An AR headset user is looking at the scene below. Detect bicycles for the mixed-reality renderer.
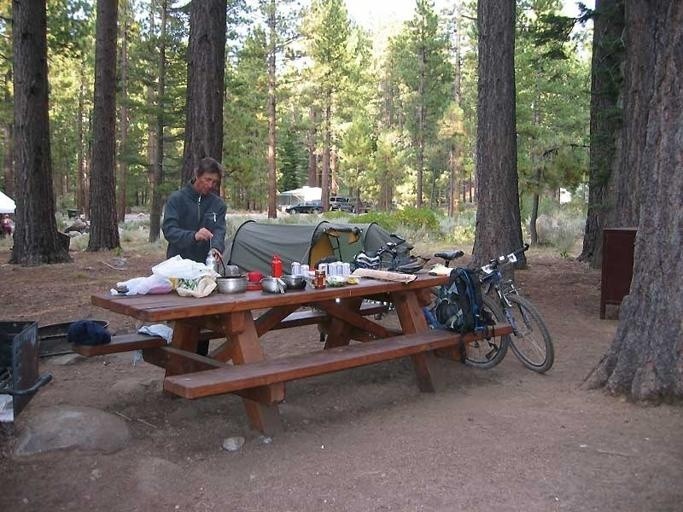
[317,233,555,374]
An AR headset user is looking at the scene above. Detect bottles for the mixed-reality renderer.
[271,256,282,277]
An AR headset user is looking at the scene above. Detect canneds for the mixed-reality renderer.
[313,270,326,290]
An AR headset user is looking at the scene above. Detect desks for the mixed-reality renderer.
[92,271,451,438]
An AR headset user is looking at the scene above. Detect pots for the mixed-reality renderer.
[216,276,247,294]
[260,276,287,294]
[281,274,307,290]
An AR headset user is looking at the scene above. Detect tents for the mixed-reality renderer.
[218,219,415,277]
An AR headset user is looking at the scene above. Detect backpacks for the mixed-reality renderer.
[435,267,484,330]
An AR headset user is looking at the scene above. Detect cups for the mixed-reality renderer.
[301,263,309,274]
[290,261,300,274]
[317,261,351,276]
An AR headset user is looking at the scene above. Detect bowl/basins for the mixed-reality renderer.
[344,274,360,284]
[325,277,347,287]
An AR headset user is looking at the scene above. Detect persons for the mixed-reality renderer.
[162,156,228,355]
[3,215,13,235]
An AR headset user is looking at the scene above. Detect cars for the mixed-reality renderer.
[286,196,374,215]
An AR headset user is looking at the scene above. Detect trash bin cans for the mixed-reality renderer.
[600,229,639,320]
[66,209,80,219]
